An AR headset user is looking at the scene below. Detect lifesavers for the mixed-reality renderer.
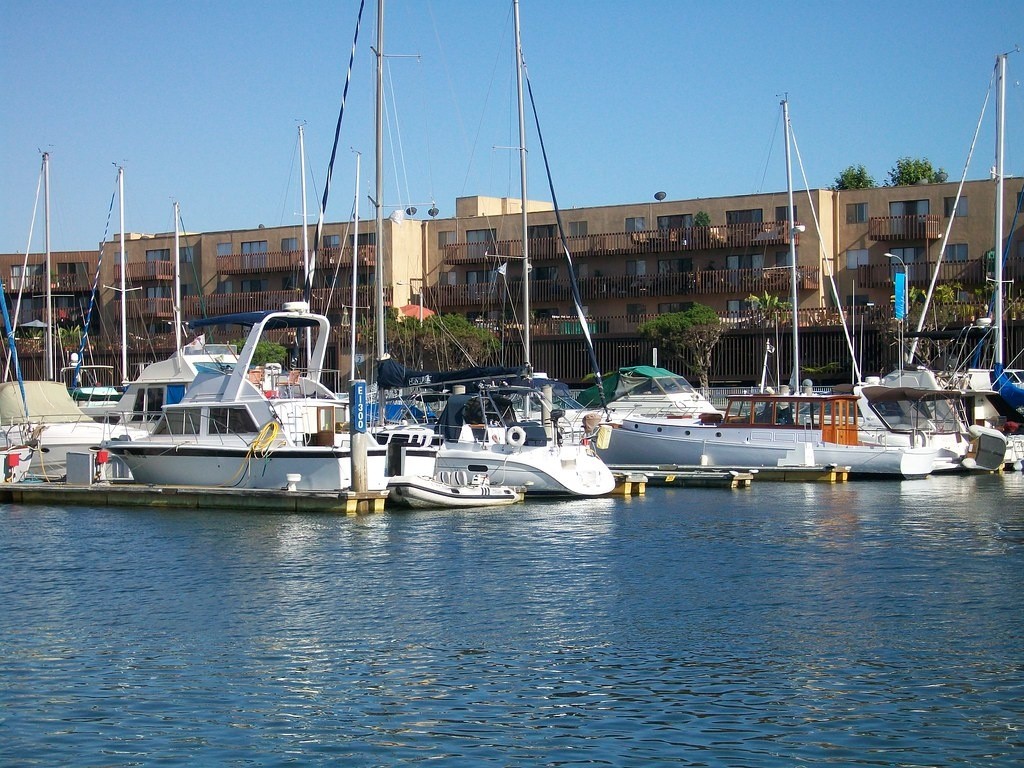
[507,426,526,446]
[910,430,928,447]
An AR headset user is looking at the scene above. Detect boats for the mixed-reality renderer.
[1,0,1024,510]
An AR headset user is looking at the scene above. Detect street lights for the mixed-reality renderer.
[884,252,909,335]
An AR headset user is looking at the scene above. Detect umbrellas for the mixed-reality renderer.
[20,319,53,331]
